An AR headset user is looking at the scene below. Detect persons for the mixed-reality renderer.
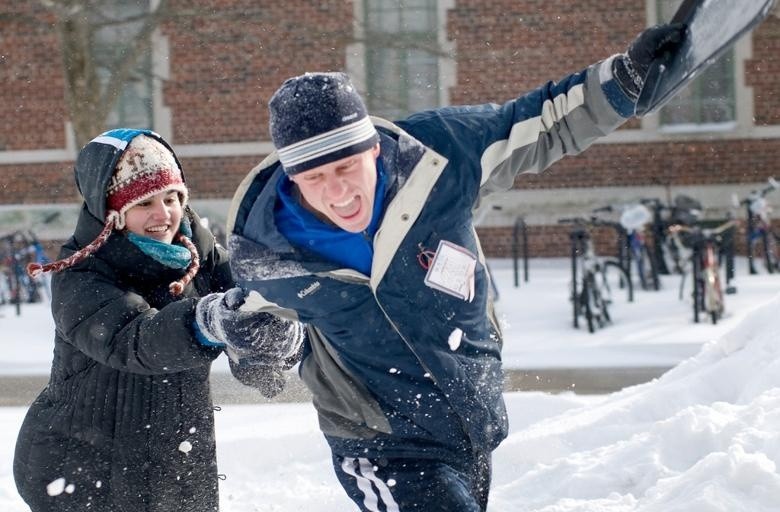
[224,0,767,511]
[620,198,693,276]
[1,229,54,304]
[11,125,305,511]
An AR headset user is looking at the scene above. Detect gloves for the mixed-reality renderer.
[195,288,305,398]
[615,22,693,113]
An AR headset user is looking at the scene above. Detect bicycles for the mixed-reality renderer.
[594,180,779,324]
[0,212,63,314]
[558,205,632,333]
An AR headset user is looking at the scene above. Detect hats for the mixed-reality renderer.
[268,70,381,178]
[28,134,201,295]
